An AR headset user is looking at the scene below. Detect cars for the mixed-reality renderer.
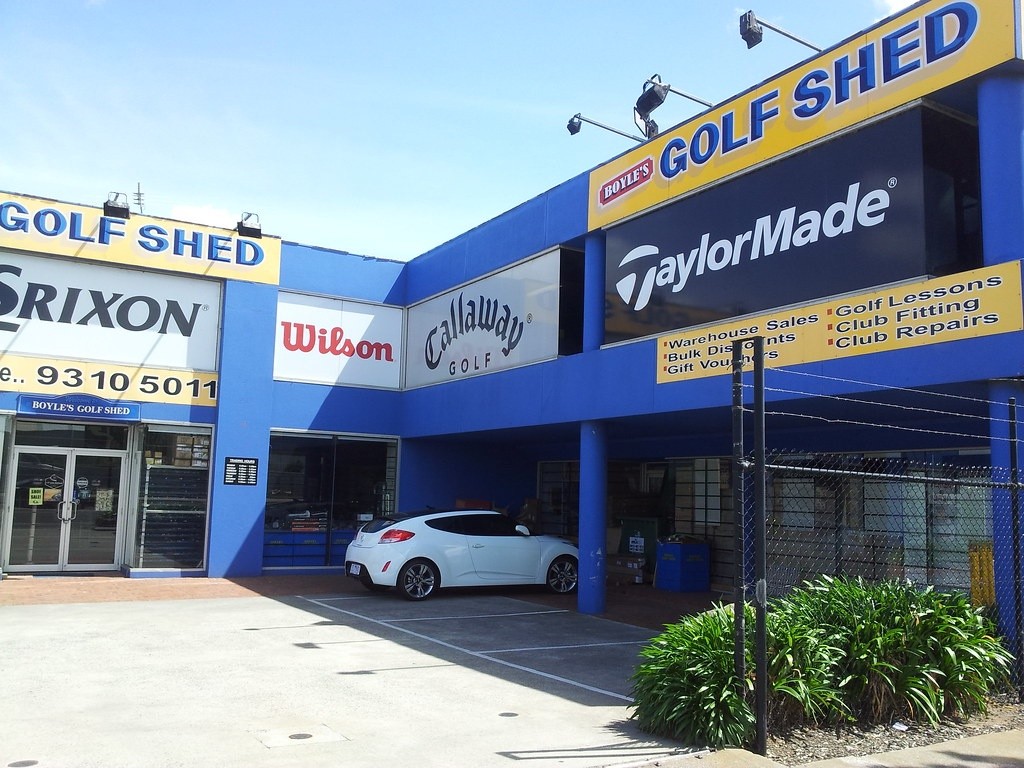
[344,508,578,601]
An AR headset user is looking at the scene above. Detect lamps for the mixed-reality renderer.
[635,74,713,119]
[103,191,131,220]
[567,113,646,143]
[739,10,822,52]
[633,106,658,139]
[233,212,262,239]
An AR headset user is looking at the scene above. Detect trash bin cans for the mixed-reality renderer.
[614,509,669,556]
[655,541,712,592]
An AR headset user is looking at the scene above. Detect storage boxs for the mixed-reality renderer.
[142,435,210,468]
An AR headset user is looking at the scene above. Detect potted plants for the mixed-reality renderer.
[887,546,904,582]
